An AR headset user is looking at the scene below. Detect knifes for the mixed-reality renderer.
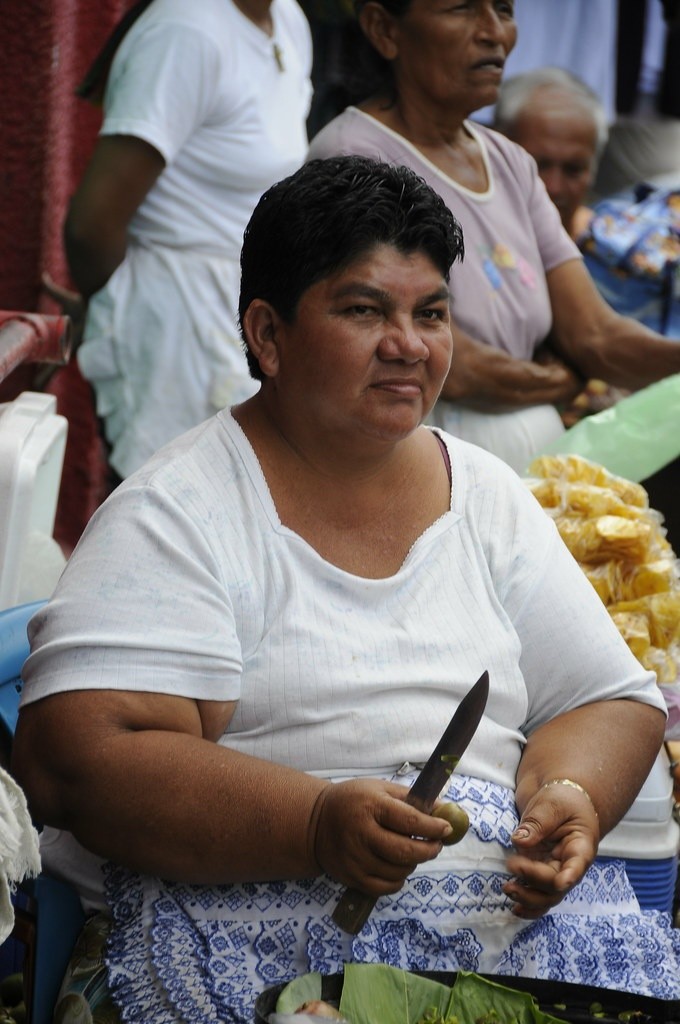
[332,669,490,935]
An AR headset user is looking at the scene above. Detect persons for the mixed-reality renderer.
[494,67,610,239]
[11,154,680,1024]
[615,0,680,120]
[307,0,680,477]
[42,0,315,492]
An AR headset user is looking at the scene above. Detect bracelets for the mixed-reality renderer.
[544,778,598,817]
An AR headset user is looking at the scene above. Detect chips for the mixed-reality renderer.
[525,454,679,688]
